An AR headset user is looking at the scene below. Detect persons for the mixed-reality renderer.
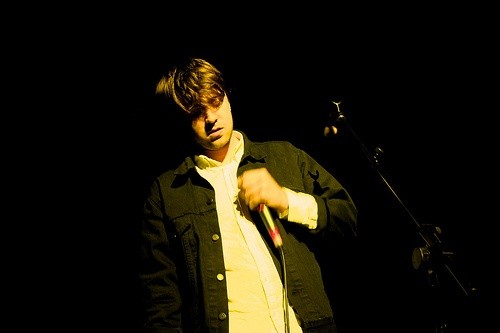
[135,56,358,333]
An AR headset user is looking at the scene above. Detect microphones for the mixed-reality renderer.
[237,165,284,249]
